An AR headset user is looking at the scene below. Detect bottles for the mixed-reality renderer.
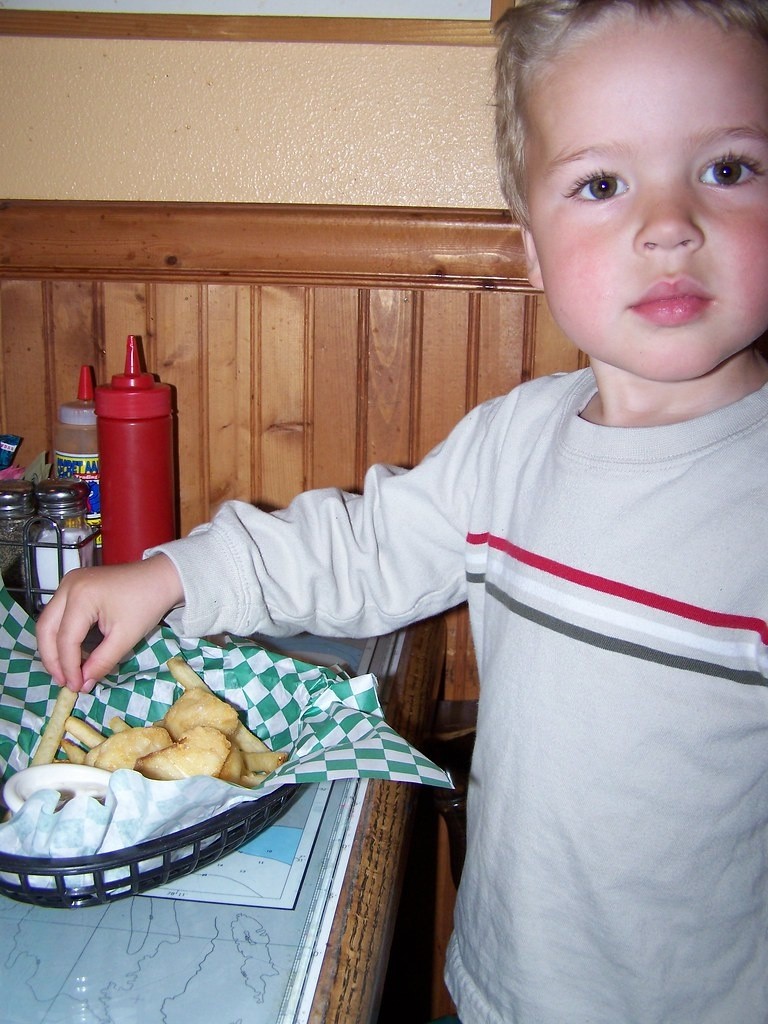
[0,479,40,617]
[50,363,111,566]
[94,333,176,566]
[32,478,96,613]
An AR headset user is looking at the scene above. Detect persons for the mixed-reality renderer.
[38,3,765,1024]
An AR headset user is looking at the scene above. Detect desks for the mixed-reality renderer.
[1,615,448,1024]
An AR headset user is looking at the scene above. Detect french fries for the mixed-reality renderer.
[0,657,288,826]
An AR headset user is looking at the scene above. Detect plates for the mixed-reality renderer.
[3,762,114,814]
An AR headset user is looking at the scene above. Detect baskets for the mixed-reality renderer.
[1,777,303,907]
